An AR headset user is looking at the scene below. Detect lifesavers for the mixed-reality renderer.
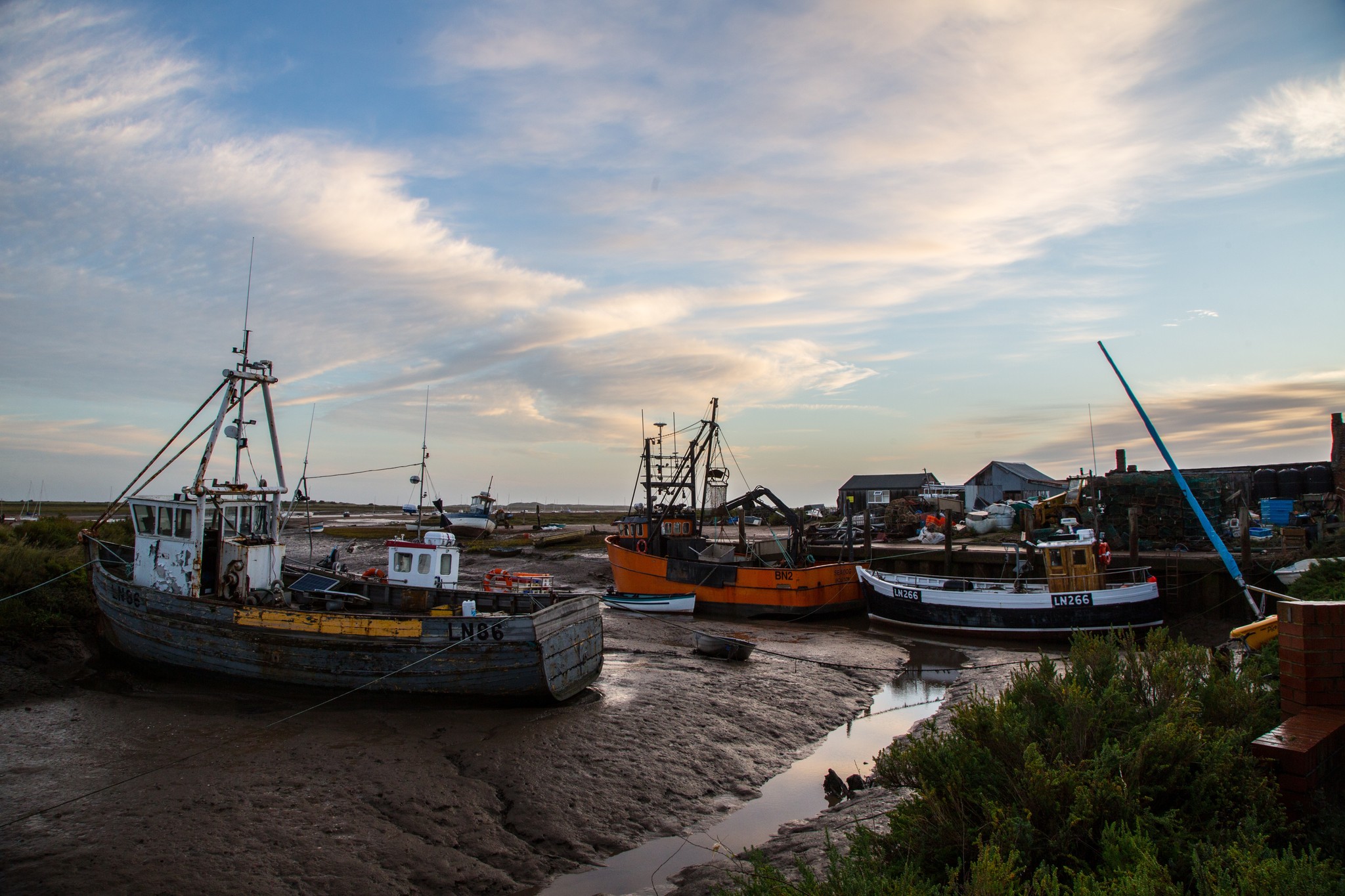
[1099,542,1105,566]
[483,568,513,594]
[636,539,647,554]
[1102,542,1111,566]
[361,568,388,584]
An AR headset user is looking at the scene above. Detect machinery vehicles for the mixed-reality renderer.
[1020,469,1097,531]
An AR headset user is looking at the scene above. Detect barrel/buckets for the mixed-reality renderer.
[462,600,476,617]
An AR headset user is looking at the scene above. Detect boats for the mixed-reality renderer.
[459,495,463,514]
[404,522,444,530]
[304,510,314,517]
[432,492,444,516]
[344,510,350,517]
[495,493,631,514]
[300,521,324,527]
[488,547,522,556]
[77,239,606,702]
[423,513,430,516]
[305,526,324,532]
[691,629,757,660]
[442,476,497,538]
[270,499,288,518]
[604,393,869,620]
[1,517,15,520]
[532,529,586,548]
[279,374,574,595]
[548,522,566,528]
[857,517,1164,640]
[600,584,697,615]
[20,480,46,521]
